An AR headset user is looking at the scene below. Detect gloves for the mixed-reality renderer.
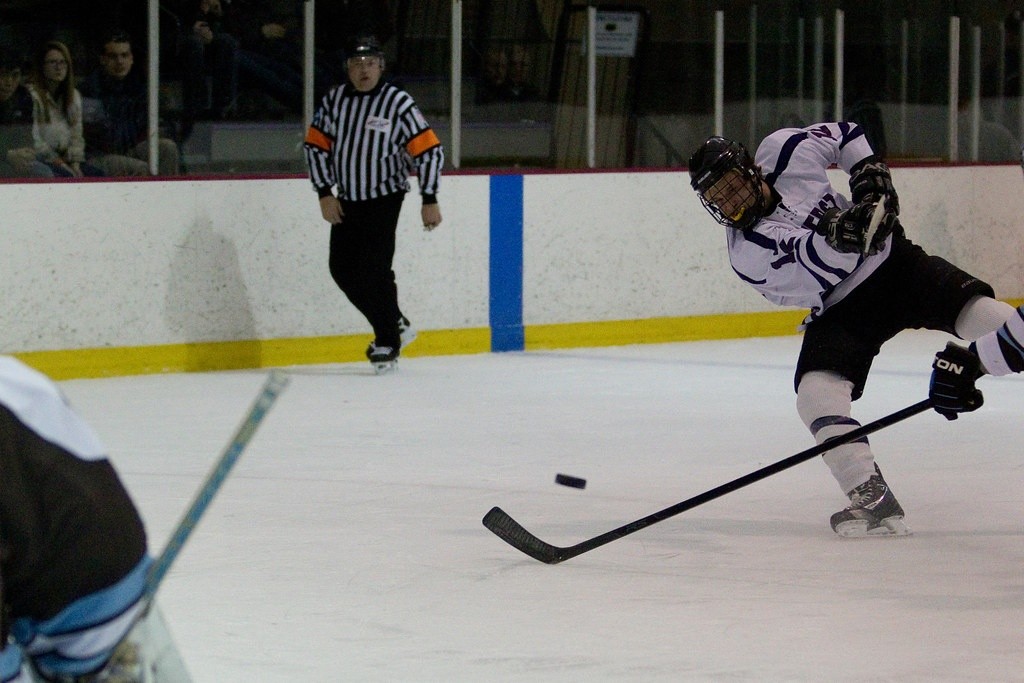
[850,155,901,217]
[929,342,984,421]
[817,206,897,257]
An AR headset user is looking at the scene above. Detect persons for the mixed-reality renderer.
[929,303,1024,421]
[184,0,395,129]
[302,36,444,361]
[76,32,182,177]
[0,44,53,178]
[484,45,532,107]
[24,40,107,177]
[686,120,1016,538]
[0,352,154,683]
[822,100,887,164]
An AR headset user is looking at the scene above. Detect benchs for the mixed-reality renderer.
[184,118,553,171]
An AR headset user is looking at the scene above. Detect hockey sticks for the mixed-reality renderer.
[481,385,980,566]
[147,369,290,588]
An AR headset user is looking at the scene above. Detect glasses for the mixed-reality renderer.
[43,59,68,68]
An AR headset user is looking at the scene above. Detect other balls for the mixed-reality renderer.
[555,473,586,489]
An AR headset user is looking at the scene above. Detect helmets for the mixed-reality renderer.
[343,36,385,72]
[689,135,765,230]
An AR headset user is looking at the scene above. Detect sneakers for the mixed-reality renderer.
[366,313,416,359]
[370,343,399,374]
[830,461,913,538]
